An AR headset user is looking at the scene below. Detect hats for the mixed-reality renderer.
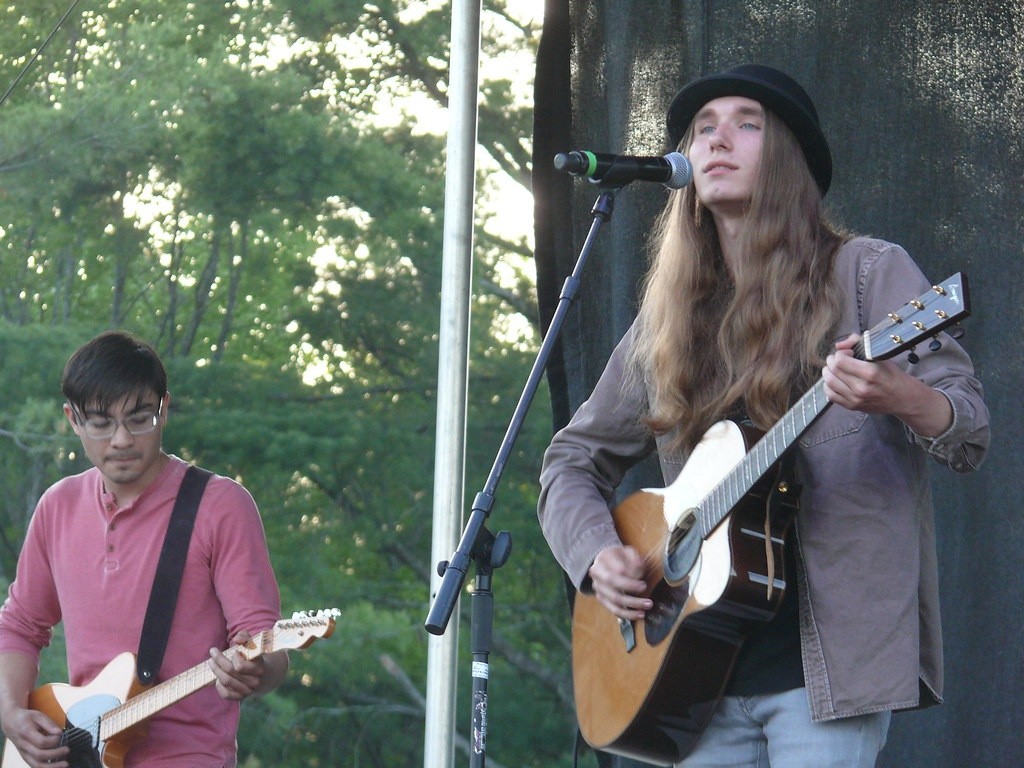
[668,65,834,205]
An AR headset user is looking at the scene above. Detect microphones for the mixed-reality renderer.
[553,150,693,190]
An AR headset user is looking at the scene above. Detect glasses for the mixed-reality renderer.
[66,392,168,439]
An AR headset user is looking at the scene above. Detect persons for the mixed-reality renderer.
[536,62,992,768]
[0,331,289,768]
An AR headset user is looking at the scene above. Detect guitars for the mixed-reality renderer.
[1,603,341,768]
[571,273,970,768]
